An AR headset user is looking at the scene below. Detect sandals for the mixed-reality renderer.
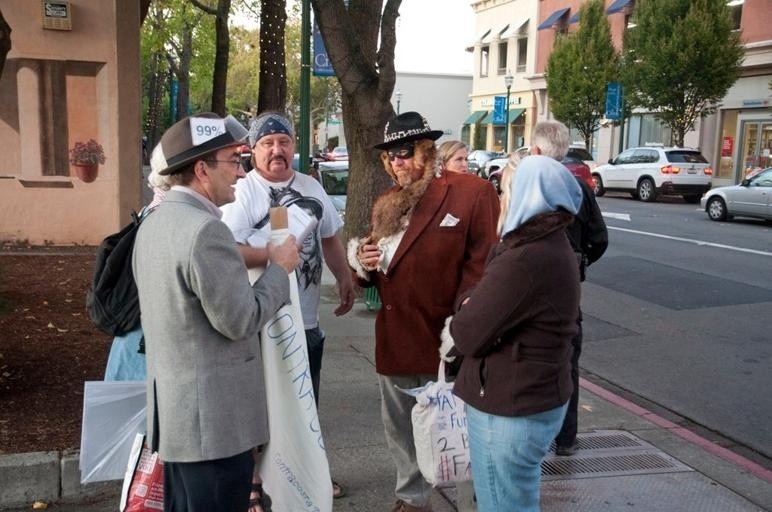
[249,483,263,512]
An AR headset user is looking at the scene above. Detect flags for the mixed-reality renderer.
[606,83,621,119]
[493,97,507,125]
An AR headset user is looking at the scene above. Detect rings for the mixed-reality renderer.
[342,301,347,305]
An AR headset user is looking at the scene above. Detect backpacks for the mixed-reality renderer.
[85,205,157,337]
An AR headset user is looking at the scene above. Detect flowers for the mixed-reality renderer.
[70,138,106,167]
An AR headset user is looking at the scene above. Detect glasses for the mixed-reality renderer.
[206,159,241,172]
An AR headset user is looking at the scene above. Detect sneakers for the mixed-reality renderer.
[556,438,579,456]
[393,498,432,512]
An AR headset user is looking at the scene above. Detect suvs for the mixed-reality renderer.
[589,140,713,203]
[483,146,595,180]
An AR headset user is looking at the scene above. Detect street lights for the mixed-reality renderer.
[503,68,515,154]
[394,87,402,115]
[147,54,178,163]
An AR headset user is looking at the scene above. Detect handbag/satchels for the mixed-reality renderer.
[119,433,164,512]
[394,380,472,487]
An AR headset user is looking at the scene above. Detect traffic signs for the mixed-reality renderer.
[327,118,343,125]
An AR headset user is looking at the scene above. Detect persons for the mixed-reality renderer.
[103,140,181,384]
[348,110,505,512]
[528,120,609,458]
[221,110,356,499]
[446,150,584,512]
[438,140,470,175]
[129,109,301,512]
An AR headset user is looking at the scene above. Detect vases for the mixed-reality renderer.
[71,159,98,181]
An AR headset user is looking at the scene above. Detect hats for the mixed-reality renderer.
[159,112,246,176]
[373,111,443,149]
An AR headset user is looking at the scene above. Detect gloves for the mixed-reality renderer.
[437,316,459,363]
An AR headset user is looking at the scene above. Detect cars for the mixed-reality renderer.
[487,155,595,198]
[699,167,771,222]
[325,147,349,161]
[309,161,349,218]
[466,150,508,179]
[238,152,313,174]
